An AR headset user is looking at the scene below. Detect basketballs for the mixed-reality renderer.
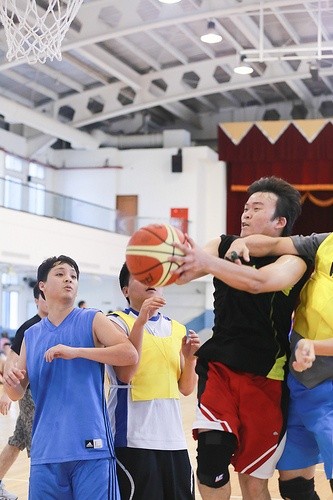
[125,223,188,287]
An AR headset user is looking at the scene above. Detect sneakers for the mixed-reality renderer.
[0,482,19,500]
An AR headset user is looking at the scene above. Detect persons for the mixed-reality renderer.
[223,230,333,500]
[166,175,315,500]
[103,262,201,500]
[0,254,139,500]
